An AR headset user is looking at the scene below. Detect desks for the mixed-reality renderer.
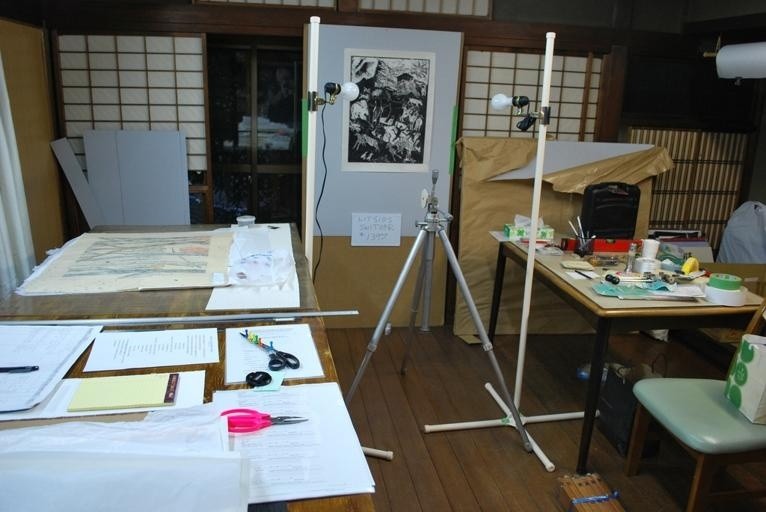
[1,223,376,512]
[489,240,765,475]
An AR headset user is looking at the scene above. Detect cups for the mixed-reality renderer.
[641,239,660,260]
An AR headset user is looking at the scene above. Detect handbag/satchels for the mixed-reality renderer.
[724,333,766,425]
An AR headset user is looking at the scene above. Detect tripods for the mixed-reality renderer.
[344,169,533,454]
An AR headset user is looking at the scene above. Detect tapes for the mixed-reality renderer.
[704,273,748,306]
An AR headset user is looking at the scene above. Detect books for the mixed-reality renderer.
[604,269,648,280]
[66,374,181,413]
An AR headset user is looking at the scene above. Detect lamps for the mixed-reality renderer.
[492,93,550,131]
[307,82,359,111]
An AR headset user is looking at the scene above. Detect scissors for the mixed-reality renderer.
[240,333,299,371]
[221,408,309,433]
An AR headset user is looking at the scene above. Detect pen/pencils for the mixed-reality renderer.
[0,366,38,372]
[568,216,596,252]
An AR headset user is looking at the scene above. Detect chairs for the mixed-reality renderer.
[625,299,766,512]
[579,180,640,250]
